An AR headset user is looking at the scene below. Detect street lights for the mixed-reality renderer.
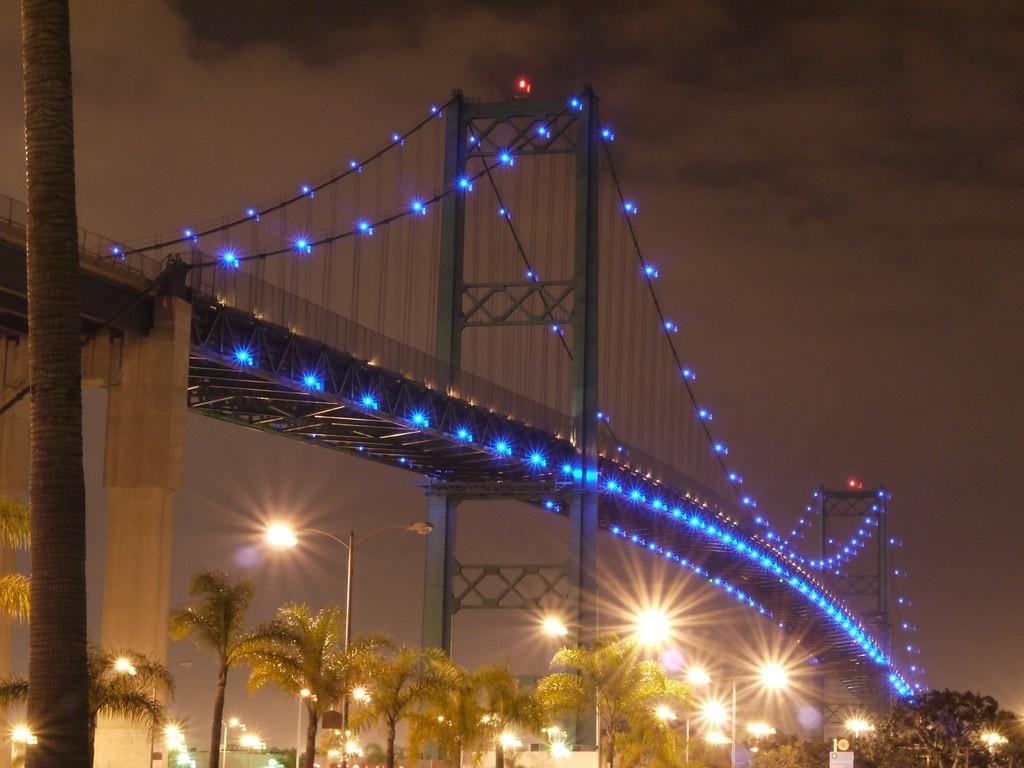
[546,613,669,768]
[213,719,239,768]
[115,658,194,768]
[656,701,727,768]
[686,664,785,768]
[296,686,310,767]
[268,518,434,767]
